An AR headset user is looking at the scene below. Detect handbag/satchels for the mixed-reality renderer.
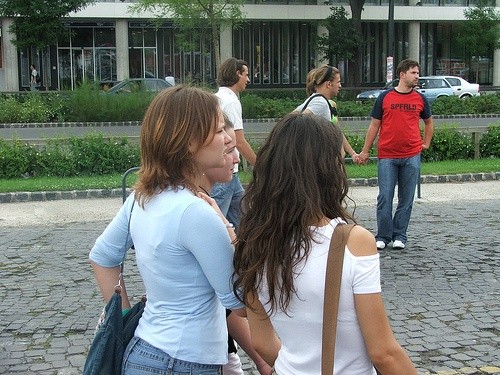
[82,284,149,375]
[31,71,42,83]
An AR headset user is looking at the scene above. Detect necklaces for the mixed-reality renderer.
[199,185,210,197]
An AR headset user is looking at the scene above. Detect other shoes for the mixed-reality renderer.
[376,240,386,249]
[392,239,405,249]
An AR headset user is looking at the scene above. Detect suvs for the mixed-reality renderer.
[356,76,458,103]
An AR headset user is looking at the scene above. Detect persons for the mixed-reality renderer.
[358,57,435,250]
[103,85,110,91]
[199,57,257,230]
[29,64,42,91]
[165,70,175,86]
[290,63,360,166]
[88,78,420,375]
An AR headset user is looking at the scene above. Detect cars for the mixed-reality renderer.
[431,76,482,101]
[97,77,174,97]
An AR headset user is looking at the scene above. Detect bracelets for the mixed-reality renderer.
[362,145,370,153]
[224,223,235,228]
[346,145,356,156]
[227,228,236,231]
[231,238,239,245]
